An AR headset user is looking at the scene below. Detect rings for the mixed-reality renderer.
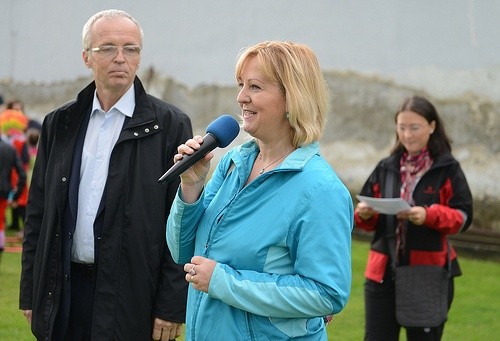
[190,265,195,275]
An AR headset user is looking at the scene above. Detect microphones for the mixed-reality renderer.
[158,114,240,184]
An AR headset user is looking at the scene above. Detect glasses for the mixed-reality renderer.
[85,45,142,57]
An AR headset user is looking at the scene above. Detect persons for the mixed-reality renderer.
[352,97,473,341]
[0,95,41,251]
[18,10,193,341]
[166,42,354,341]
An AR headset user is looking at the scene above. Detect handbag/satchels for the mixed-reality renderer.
[394,264,448,328]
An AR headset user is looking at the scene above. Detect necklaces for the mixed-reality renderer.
[260,147,292,173]
